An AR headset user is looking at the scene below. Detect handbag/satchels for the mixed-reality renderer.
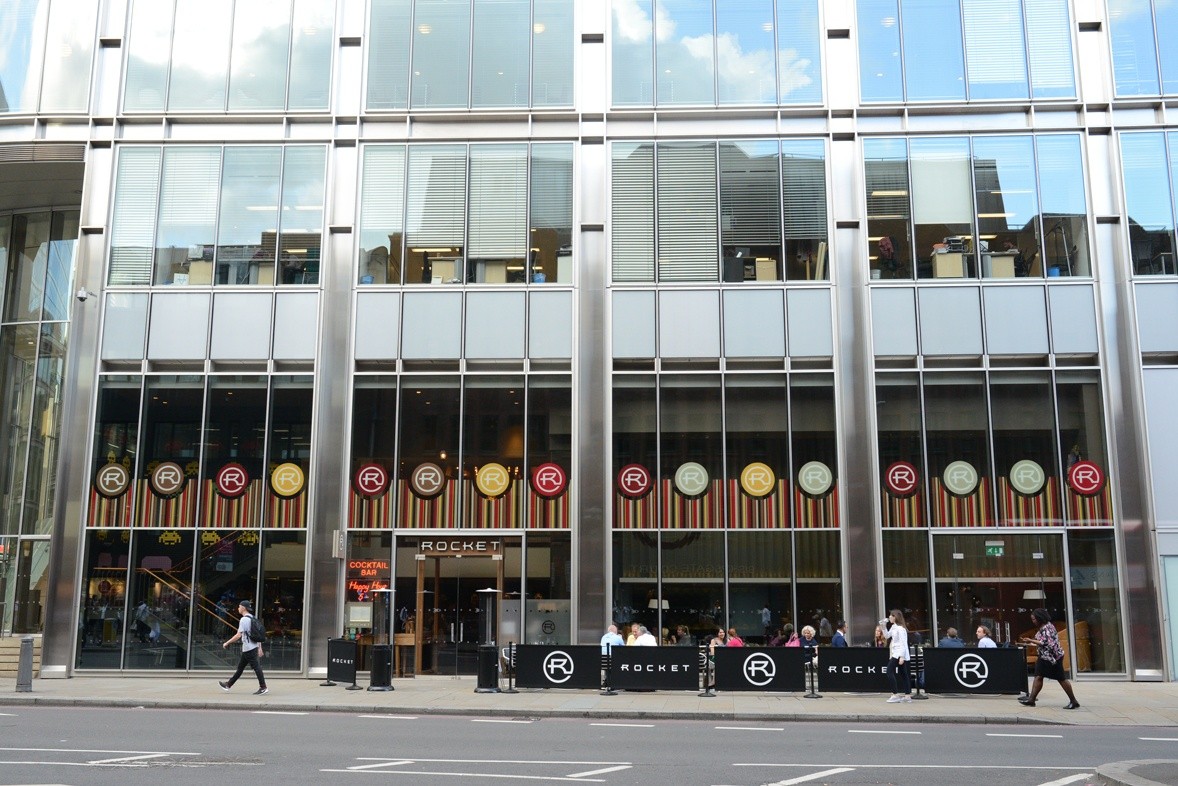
[129,619,137,630]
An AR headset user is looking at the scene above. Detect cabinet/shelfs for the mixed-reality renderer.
[367,246,399,284]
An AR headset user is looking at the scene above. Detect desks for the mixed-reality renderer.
[980,251,1020,278]
[484,259,507,283]
[741,256,757,280]
[427,256,464,284]
[258,261,284,284]
[1151,251,1173,274]
[188,258,213,285]
[756,259,778,281]
[931,252,974,278]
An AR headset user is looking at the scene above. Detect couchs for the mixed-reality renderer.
[1019,620,1094,674]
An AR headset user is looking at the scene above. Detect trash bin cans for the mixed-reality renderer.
[366,643,394,692]
[474,644,501,694]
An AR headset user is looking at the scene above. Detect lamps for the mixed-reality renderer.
[647,599,670,626]
[1022,590,1047,609]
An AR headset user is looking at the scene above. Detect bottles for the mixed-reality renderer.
[879,618,889,624]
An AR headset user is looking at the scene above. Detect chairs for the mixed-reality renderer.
[500,647,515,689]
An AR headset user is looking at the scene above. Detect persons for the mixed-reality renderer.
[218,599,269,696]
[879,609,912,703]
[937,626,965,648]
[1002,240,1026,276]
[84,589,167,646]
[599,621,658,692]
[799,624,819,647]
[975,624,997,648]
[812,608,834,637]
[769,622,794,647]
[724,627,746,647]
[829,619,849,648]
[708,627,725,656]
[867,625,888,648]
[661,626,677,646]
[676,624,692,646]
[1018,608,1080,709]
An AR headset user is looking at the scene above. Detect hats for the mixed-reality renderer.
[240,601,253,611]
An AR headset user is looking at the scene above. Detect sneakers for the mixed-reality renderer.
[218,681,229,691]
[254,688,268,696]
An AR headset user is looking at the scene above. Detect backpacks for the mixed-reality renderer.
[244,615,265,642]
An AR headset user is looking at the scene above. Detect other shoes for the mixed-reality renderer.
[1020,701,1035,707]
[1063,703,1080,709]
[887,695,900,703]
[900,697,912,703]
